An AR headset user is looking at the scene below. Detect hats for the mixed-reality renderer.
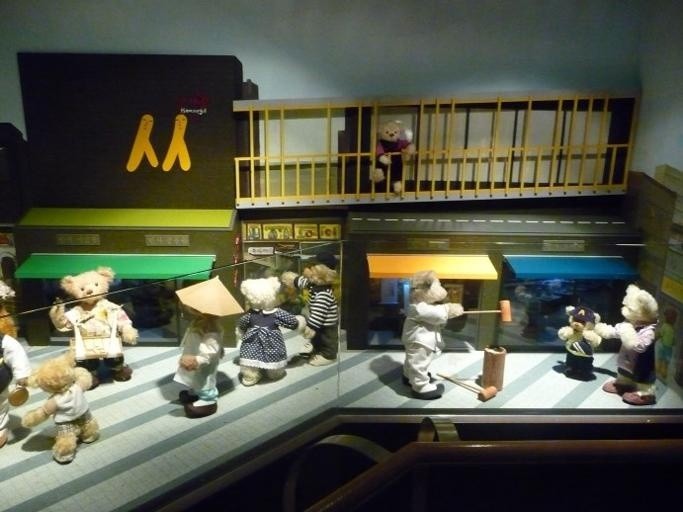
[176,275,245,317]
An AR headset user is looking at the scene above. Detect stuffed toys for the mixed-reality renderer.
[403,271,465,399]
[24,355,101,464]
[0,281,29,449]
[49,267,137,389]
[597,284,658,406]
[235,276,306,386]
[559,306,601,380]
[373,121,414,192]
[280,264,337,366]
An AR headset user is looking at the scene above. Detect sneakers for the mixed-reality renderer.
[309,353,337,366]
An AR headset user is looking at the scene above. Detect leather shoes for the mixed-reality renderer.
[185,401,217,417]
[402,372,432,386]
[411,383,444,400]
[603,381,637,393]
[180,389,199,403]
[621,391,656,406]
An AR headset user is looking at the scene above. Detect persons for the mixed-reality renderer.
[172,276,244,418]
